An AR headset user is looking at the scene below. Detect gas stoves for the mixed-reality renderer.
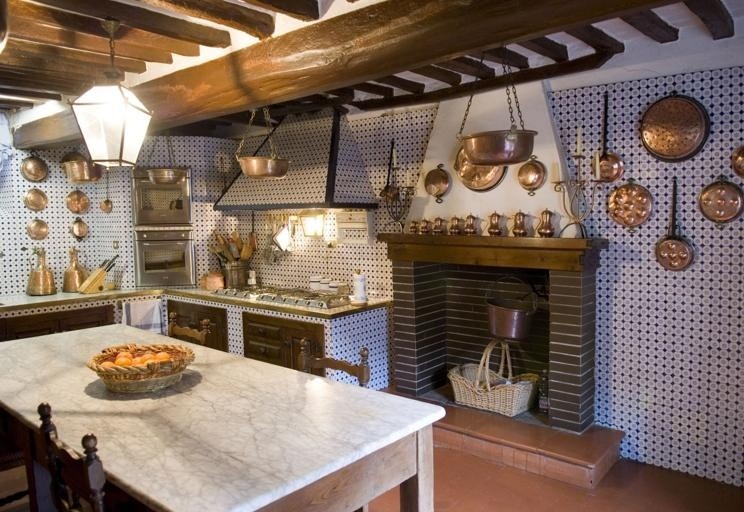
[212,284,350,311]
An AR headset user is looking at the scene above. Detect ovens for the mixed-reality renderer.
[130,168,192,225]
[133,229,197,288]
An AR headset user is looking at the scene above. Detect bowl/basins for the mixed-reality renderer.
[147,167,188,185]
[237,156,291,180]
[60,160,104,184]
[463,127,537,166]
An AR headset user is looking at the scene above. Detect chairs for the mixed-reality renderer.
[300,336,367,510]
[168,312,211,347]
[35,402,151,511]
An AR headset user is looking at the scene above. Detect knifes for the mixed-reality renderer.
[100,254,119,272]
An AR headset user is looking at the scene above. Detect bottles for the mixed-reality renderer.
[309,276,351,294]
[206,272,224,290]
[247,270,257,285]
[353,269,366,301]
[538,369,548,414]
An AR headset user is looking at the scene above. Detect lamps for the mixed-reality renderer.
[71,20,154,169]
[298,210,323,237]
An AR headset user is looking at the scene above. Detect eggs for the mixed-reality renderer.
[101,351,170,371]
[107,282,115,290]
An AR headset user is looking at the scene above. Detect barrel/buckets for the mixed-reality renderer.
[484,276,540,341]
[226,261,247,289]
[354,269,365,300]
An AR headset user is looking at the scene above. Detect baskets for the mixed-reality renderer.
[85,339,196,397]
[445,335,541,417]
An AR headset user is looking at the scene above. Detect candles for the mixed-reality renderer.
[551,162,559,182]
[576,126,583,154]
[595,152,601,179]
[393,148,397,167]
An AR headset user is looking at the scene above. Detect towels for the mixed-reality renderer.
[121,300,165,333]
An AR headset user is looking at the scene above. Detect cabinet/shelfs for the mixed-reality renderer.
[243,299,325,378]
[0,295,114,342]
[165,290,228,352]
